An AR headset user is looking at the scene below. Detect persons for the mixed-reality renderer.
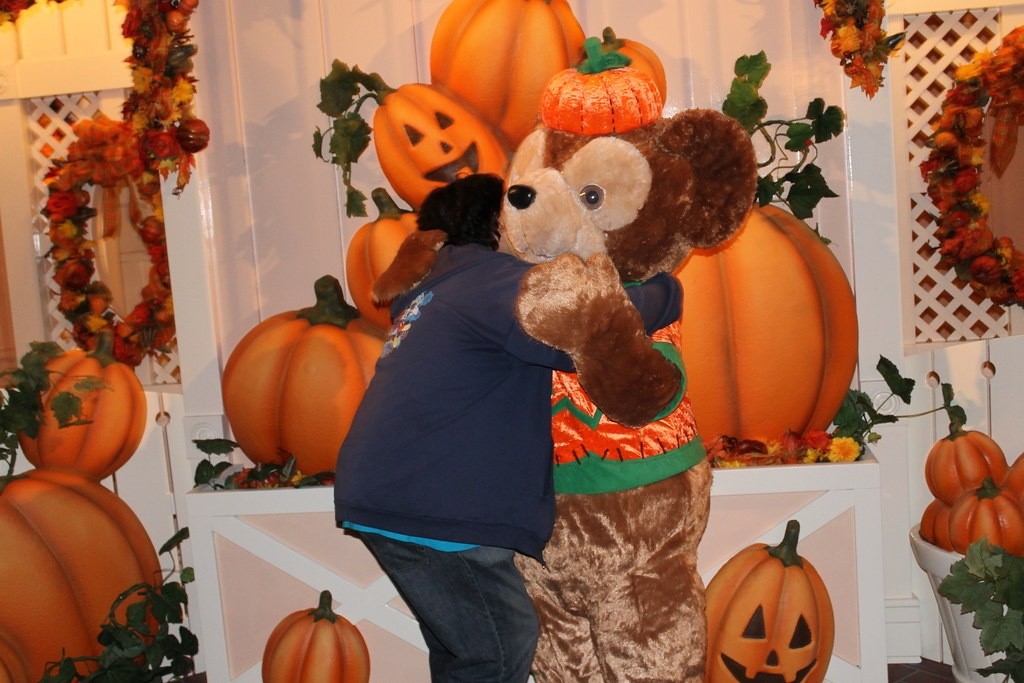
[331,173,683,683]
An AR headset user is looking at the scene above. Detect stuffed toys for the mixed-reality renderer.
[500,37,758,683]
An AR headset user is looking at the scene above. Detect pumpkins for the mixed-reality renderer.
[2,0,1023,683]
